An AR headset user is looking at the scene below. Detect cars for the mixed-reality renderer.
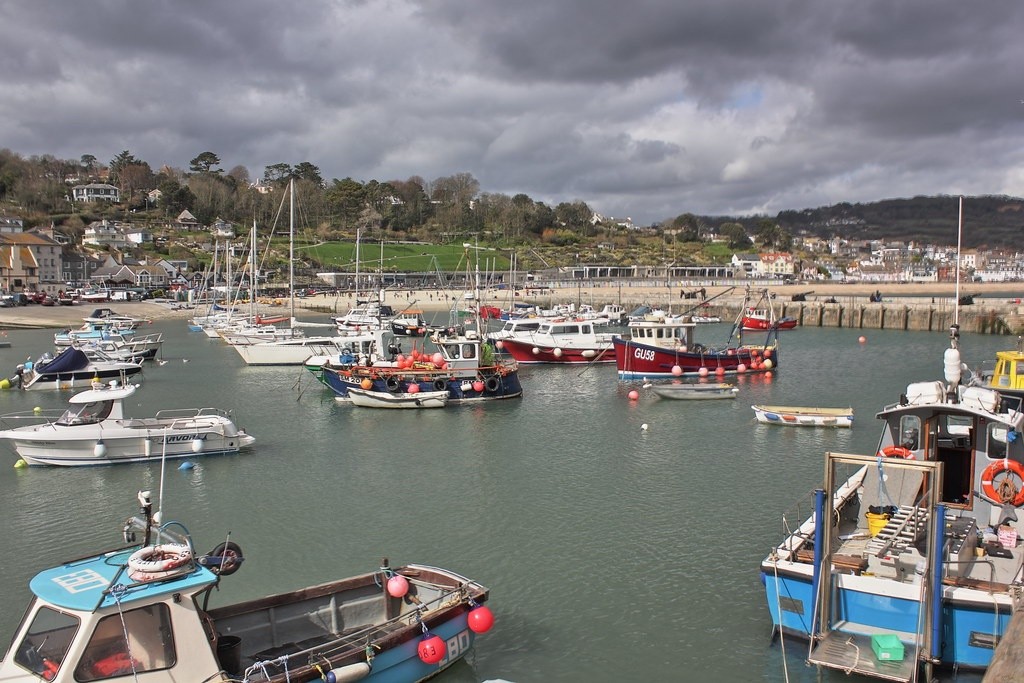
[0,285,151,308]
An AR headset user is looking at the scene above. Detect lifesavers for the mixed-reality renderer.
[485,377,500,392]
[876,447,916,460]
[386,376,399,391]
[433,378,447,391]
[128,543,192,573]
[127,563,193,582]
[341,348,352,356]
[981,459,1024,507]
[553,320,564,322]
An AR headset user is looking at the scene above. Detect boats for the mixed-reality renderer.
[1,423,495,683]
[739,306,798,331]
[0,306,166,393]
[611,284,780,378]
[346,386,451,408]
[641,377,739,399]
[965,348,1024,392]
[0,367,257,468]
[751,404,855,427]
[185,177,722,402]
[757,193,1024,683]
